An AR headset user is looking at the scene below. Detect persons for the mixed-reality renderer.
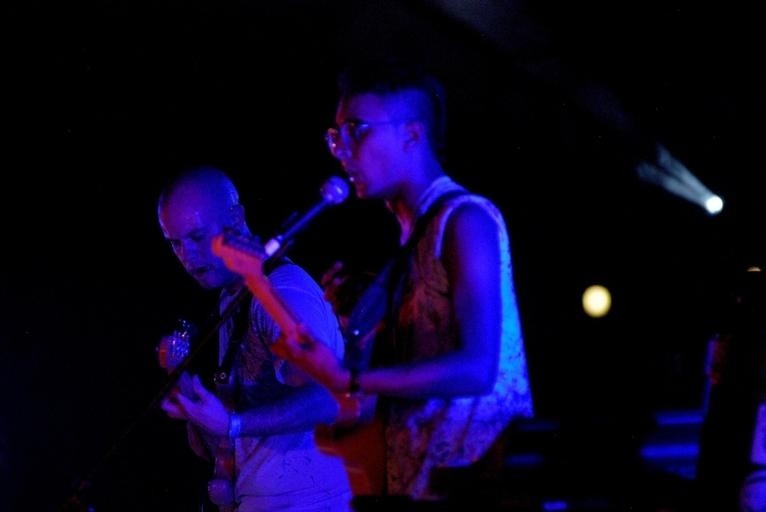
[142,165,357,511]
[258,58,536,512]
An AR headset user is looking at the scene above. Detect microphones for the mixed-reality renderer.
[264,176,351,258]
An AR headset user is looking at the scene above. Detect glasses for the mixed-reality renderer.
[327,120,394,149]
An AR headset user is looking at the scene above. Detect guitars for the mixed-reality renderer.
[211,226,433,496]
[158,329,238,511]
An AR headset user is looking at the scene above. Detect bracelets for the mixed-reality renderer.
[226,410,244,438]
[347,369,359,395]
